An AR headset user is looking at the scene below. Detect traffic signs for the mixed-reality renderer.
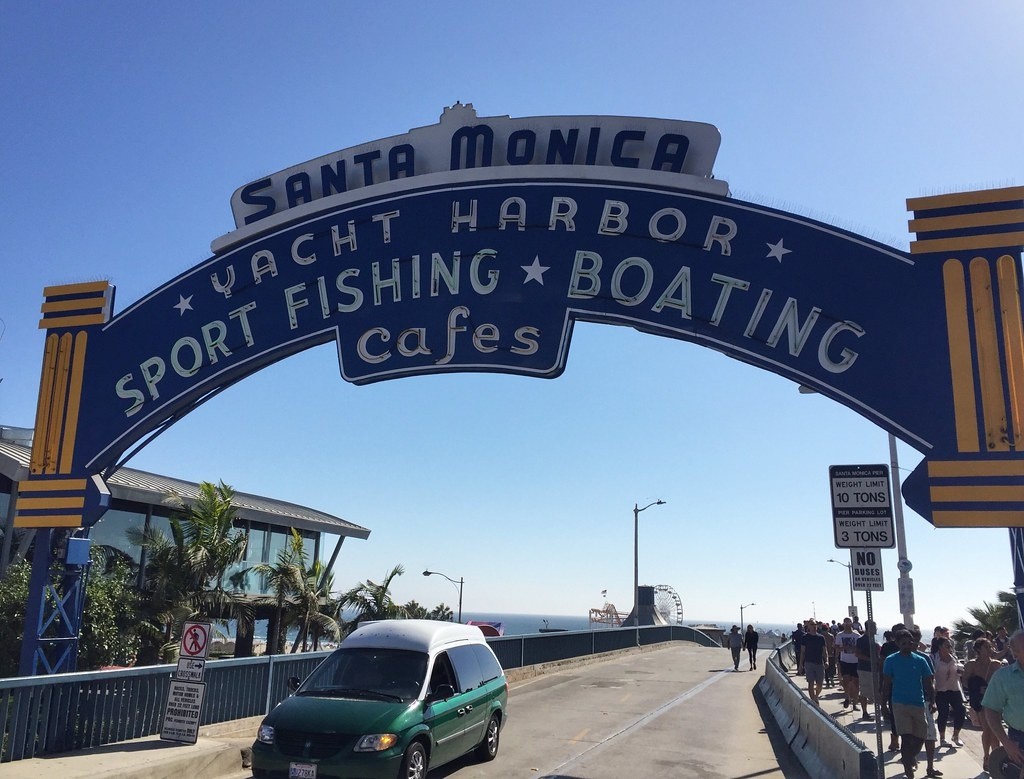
[177,657,206,683]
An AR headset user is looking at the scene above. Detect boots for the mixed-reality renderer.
[888,734,899,750]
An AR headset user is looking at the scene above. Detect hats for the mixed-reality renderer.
[821,624,828,629]
[731,625,741,632]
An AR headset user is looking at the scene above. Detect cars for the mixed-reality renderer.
[252,619,510,779]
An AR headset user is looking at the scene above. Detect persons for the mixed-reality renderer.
[743,625,759,671]
[790,616,883,721]
[728,625,745,672]
[877,623,1024,779]
[781,633,787,644]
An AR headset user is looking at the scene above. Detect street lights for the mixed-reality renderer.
[633,500,666,626]
[827,559,855,623]
[422,570,464,623]
[740,603,755,637]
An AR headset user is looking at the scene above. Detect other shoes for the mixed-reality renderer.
[749,668,753,671]
[844,700,849,708]
[927,769,943,776]
[826,682,830,688]
[983,758,990,770]
[951,735,963,746]
[853,705,859,711]
[901,759,914,779]
[862,713,874,721]
[753,663,756,670]
[941,739,952,747]
[831,682,836,686]
